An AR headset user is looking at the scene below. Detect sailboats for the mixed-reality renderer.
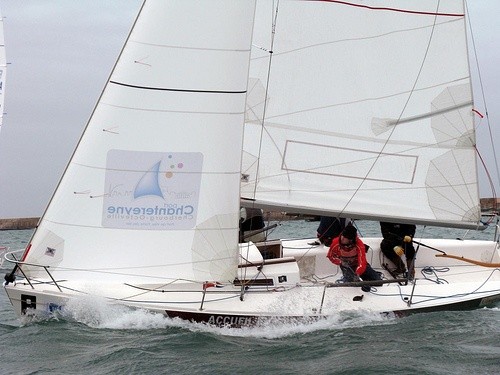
[2,0,500,330]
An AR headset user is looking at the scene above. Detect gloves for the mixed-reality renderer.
[393,246,404,255]
[403,236,411,242]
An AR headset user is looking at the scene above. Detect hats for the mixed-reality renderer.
[342,226,358,241]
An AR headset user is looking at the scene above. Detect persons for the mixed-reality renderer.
[239,206,264,243]
[380,221,417,281]
[327,224,384,287]
[317,215,352,247]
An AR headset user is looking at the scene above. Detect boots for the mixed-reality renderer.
[406,260,414,276]
[392,258,405,275]
